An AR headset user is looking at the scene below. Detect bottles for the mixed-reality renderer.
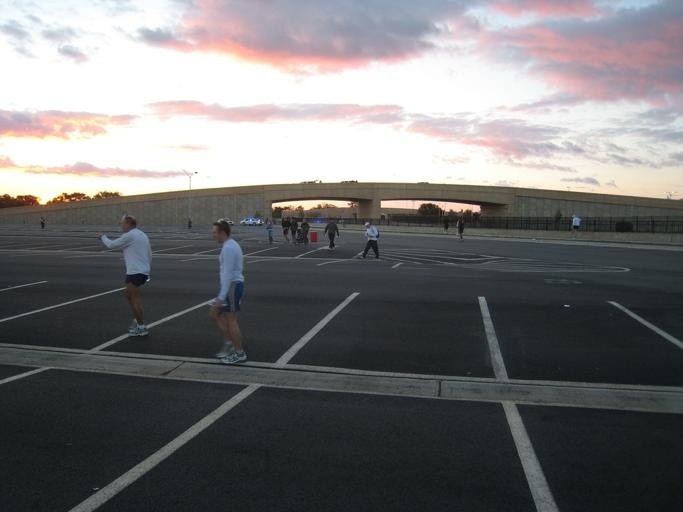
[207,299,228,307]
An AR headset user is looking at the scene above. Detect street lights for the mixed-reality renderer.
[182,169,198,228]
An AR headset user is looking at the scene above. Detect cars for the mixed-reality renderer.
[216,218,234,226]
[238,218,263,226]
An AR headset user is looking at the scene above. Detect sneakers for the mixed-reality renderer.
[215,342,238,359]
[128,318,150,337]
[221,349,248,365]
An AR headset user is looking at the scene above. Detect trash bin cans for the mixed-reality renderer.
[310,231,318,242]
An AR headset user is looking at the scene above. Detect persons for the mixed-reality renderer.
[456,215,465,240]
[209,220,248,365]
[360,222,379,259]
[95,215,152,336]
[324,220,340,252]
[265,215,310,247]
[443,216,450,232]
[188,217,192,231]
[40,217,45,229]
[572,214,581,239]
